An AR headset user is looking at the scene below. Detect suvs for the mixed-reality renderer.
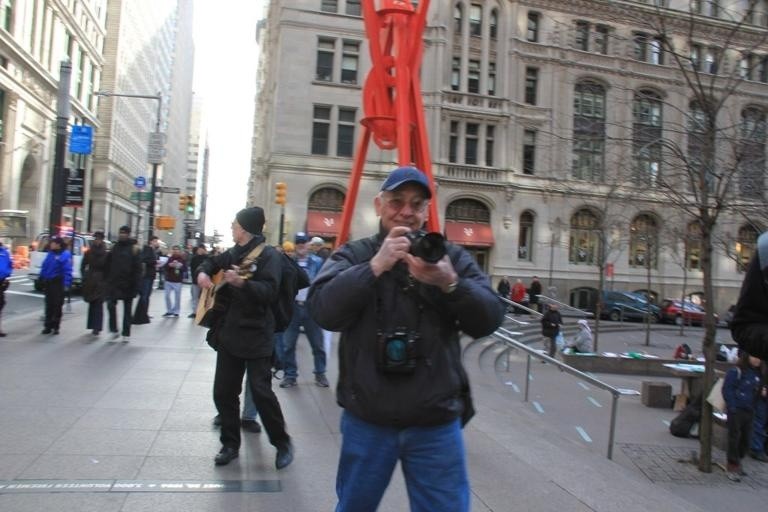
[659,296,720,327]
[597,287,662,325]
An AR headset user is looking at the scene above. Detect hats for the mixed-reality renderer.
[309,237,324,244]
[295,232,307,241]
[282,241,295,251]
[237,207,265,234]
[381,167,433,197]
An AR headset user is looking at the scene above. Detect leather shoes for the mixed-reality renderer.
[215,445,238,464]
[276,443,293,469]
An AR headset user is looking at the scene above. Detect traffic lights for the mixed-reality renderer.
[186,194,194,215]
[179,195,185,211]
[273,181,288,206]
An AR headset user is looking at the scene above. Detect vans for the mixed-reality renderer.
[28,229,119,291]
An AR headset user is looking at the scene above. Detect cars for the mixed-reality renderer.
[722,301,737,326]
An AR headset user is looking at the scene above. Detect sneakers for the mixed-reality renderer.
[280,378,296,388]
[727,471,741,482]
[748,448,768,462]
[242,419,261,433]
[316,373,329,387]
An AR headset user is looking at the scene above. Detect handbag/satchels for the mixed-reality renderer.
[706,377,728,416]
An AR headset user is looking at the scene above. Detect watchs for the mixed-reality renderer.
[443,280,461,296]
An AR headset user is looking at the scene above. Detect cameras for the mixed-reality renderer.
[376,325,423,377]
[403,230,446,263]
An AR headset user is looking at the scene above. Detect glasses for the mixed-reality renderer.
[382,195,428,213]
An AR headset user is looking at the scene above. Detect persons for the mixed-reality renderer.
[305,167,505,511]
[508,277,524,317]
[496,274,511,314]
[0,241,13,337]
[212,236,329,430]
[746,360,768,463]
[722,350,768,482]
[192,206,295,469]
[540,305,566,363]
[730,229,767,364]
[560,319,593,353]
[35,227,222,342]
[525,276,542,320]
